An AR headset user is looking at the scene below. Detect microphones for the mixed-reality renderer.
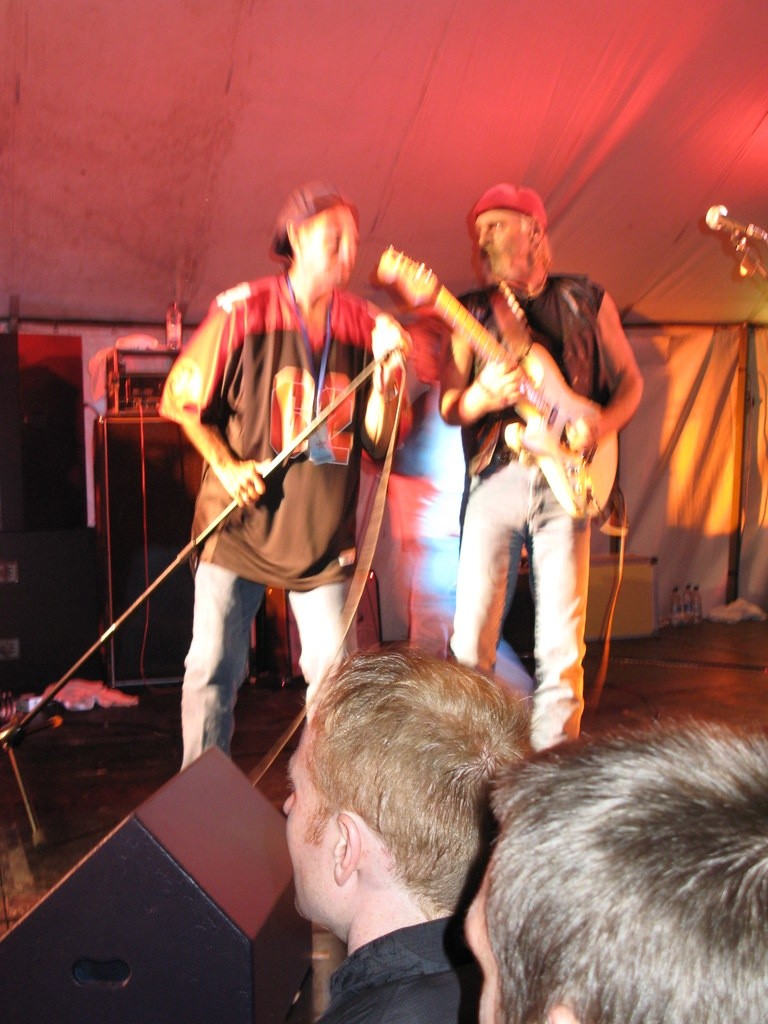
[706,205,768,245]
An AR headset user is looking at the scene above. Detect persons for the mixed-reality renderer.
[457,717,768,1024]
[156,176,647,771]
[283,639,537,1024]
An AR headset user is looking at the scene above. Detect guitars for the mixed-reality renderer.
[377,245,618,520]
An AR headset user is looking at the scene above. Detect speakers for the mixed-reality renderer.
[0,744,314,1024]
[0,526,105,700]
[0,333,89,526]
[583,555,656,640]
[94,416,203,689]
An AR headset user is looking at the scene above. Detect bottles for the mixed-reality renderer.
[682,585,692,623]
[166,300,182,351]
[671,586,681,624]
[692,586,702,624]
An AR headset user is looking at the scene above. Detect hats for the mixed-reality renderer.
[272,182,359,256]
[467,183,547,235]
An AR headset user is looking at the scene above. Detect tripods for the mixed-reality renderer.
[0,354,390,849]
